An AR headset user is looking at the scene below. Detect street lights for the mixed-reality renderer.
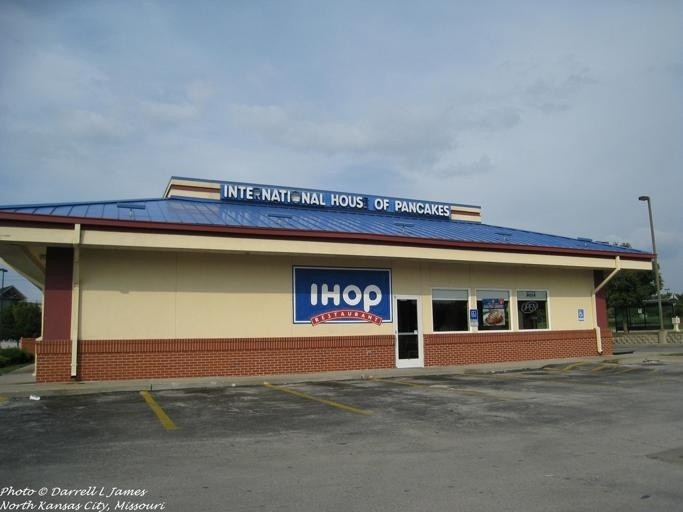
[0,268,7,288]
[636,195,665,332]
